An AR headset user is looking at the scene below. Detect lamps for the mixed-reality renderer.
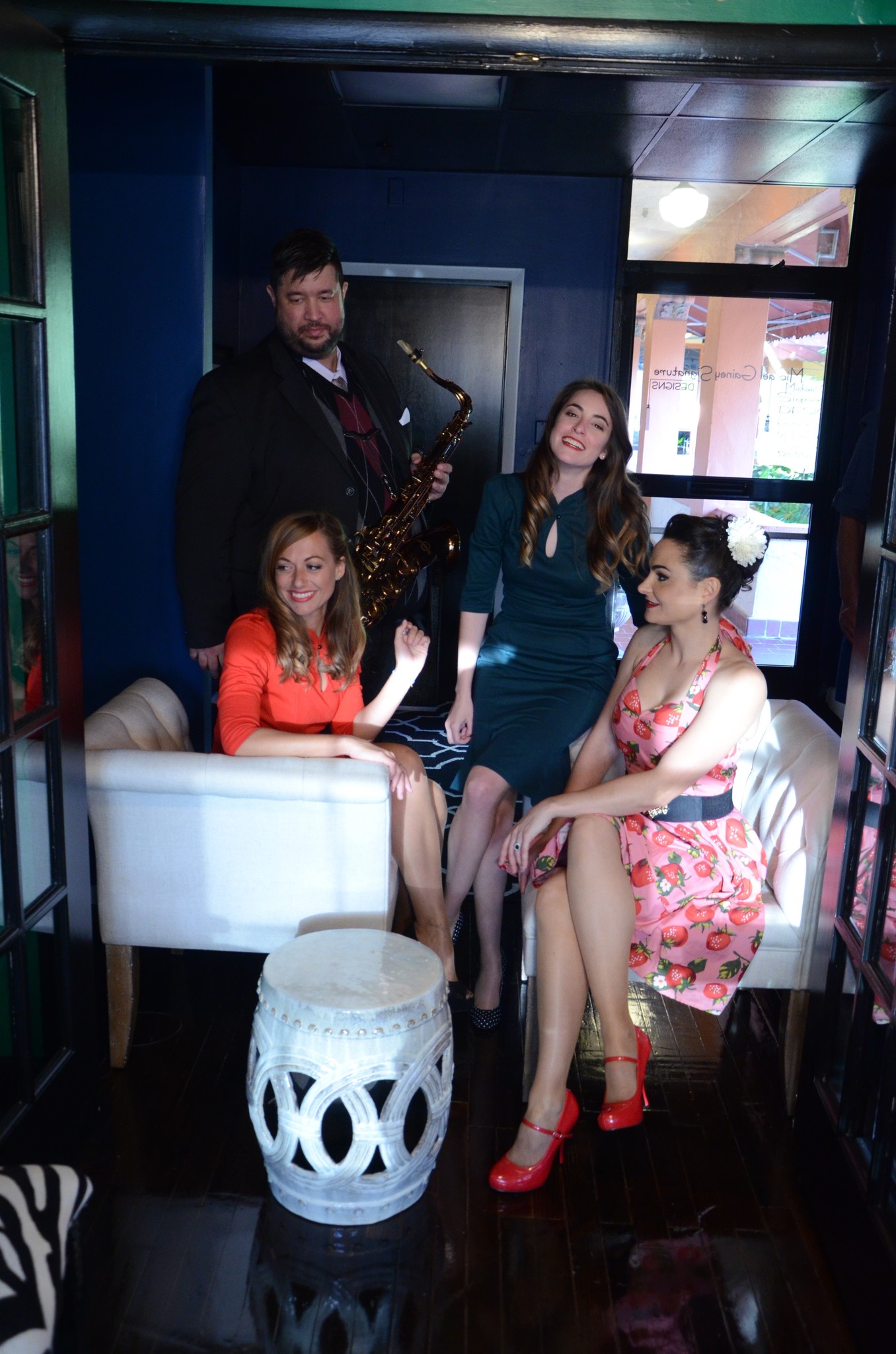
[664,178,711,229]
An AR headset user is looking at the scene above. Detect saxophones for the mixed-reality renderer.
[347,340,474,626]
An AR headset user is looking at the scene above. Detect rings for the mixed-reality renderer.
[403,630,408,634]
[513,844,523,850]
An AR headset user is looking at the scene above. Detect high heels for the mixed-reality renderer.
[489,1088,581,1192]
[471,946,508,1030]
[598,1024,650,1132]
[451,910,466,950]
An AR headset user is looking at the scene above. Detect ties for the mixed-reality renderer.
[332,376,349,393]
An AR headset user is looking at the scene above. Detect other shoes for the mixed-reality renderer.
[406,926,461,1001]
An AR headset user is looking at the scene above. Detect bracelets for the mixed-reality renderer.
[391,667,414,688]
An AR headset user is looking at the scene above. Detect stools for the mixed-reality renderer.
[237,910,455,1224]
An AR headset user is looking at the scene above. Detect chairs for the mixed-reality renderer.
[528,695,870,1117]
[89,675,404,1076]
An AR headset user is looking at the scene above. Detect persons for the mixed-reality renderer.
[6,526,83,743]
[820,626,896,1024]
[219,514,473,1011]
[488,514,768,1189]
[176,235,472,703]
[445,378,659,1029]
